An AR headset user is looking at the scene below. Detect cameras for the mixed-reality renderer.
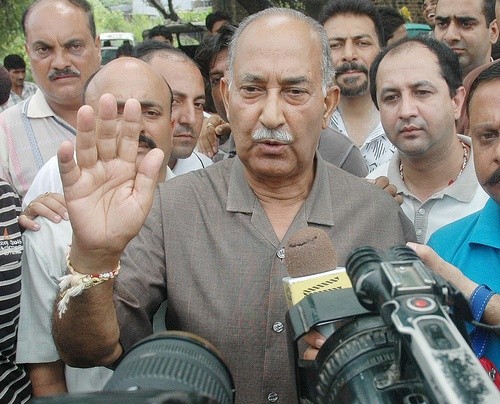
[26,330,236,404]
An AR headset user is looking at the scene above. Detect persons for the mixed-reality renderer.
[0,0,500,404]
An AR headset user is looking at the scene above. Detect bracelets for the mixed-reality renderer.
[58,250,121,316]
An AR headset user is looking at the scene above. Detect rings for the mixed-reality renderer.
[207,123,215,130]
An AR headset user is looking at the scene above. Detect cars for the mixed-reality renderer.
[99,32,136,56]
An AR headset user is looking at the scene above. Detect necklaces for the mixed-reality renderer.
[399,138,468,186]
